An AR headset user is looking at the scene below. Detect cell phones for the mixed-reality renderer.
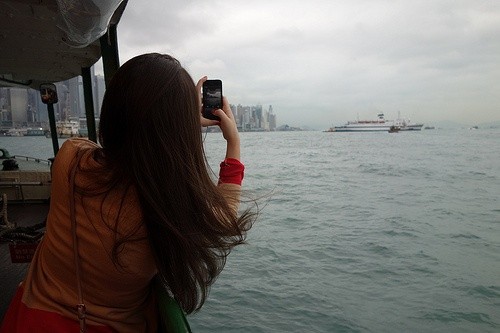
[202,80,222,121]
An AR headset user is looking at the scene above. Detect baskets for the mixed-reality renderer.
[8,239,38,263]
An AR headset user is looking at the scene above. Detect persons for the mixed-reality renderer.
[1,52,261,333]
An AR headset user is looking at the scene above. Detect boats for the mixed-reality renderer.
[329,112,424,131]
[3,118,87,139]
[388,125,400,133]
